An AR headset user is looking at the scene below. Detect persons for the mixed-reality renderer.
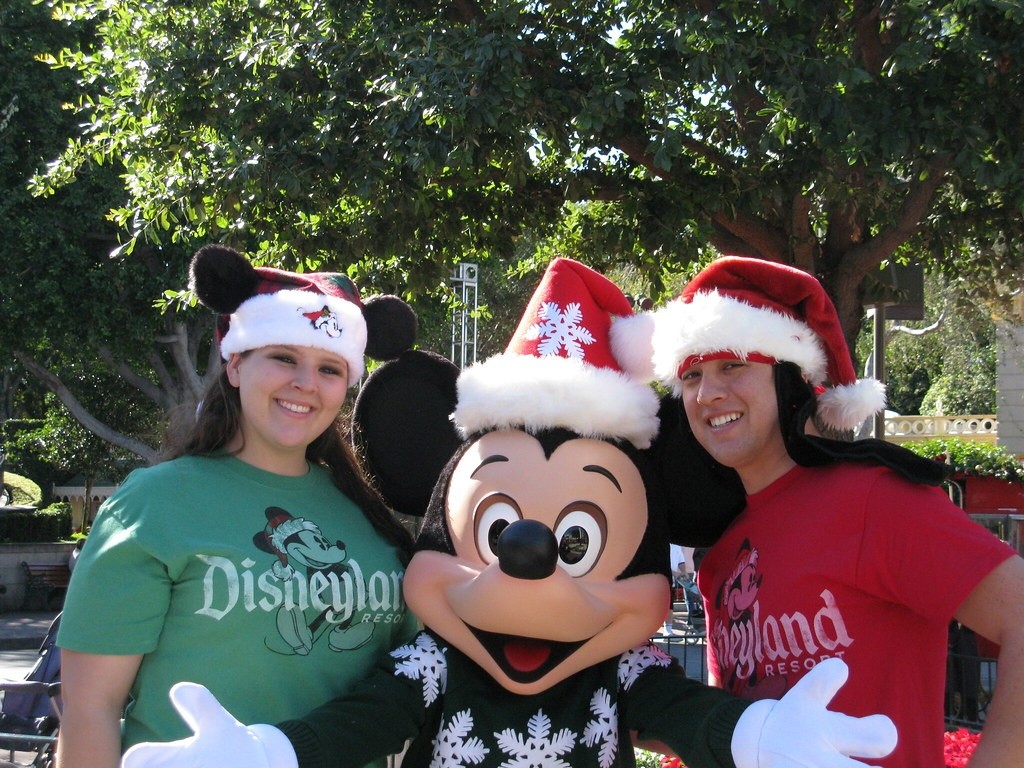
[56,245,417,768]
[664,545,689,642]
[653,257,1024,767]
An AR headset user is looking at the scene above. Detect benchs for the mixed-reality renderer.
[20,561,73,612]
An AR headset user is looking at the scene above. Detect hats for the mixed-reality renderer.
[189,244,416,389]
[651,257,887,431]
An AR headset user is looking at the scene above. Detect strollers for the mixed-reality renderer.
[677,578,707,646]
[0,608,64,761]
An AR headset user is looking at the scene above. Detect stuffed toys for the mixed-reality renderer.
[118,259,898,768]
